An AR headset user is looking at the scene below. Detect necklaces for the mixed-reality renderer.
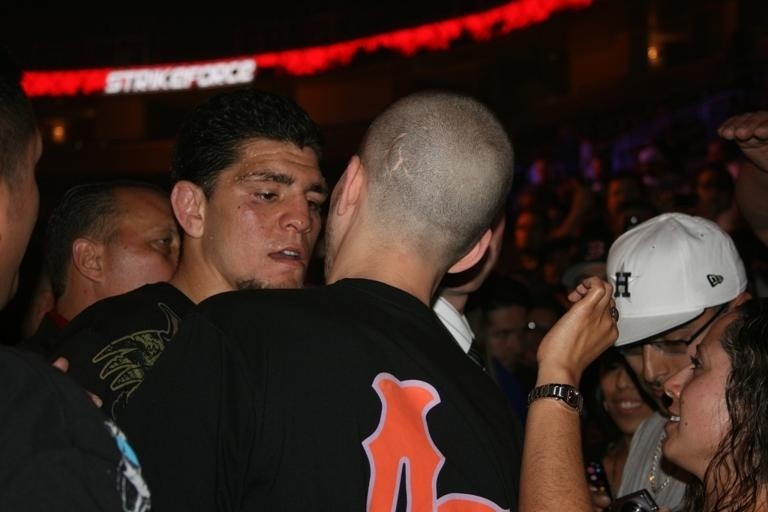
[647,429,678,495]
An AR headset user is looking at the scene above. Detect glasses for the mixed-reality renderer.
[622,306,726,354]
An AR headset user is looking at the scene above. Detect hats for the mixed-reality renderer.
[607,212,748,347]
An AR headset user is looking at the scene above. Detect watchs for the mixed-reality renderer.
[526,382,585,416]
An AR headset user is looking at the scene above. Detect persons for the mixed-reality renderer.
[116,89,527,512]
[0,77,153,512]
[517,274,768,511]
[1,110,767,384]
[592,211,753,512]
[39,81,333,420]
[585,347,657,500]
